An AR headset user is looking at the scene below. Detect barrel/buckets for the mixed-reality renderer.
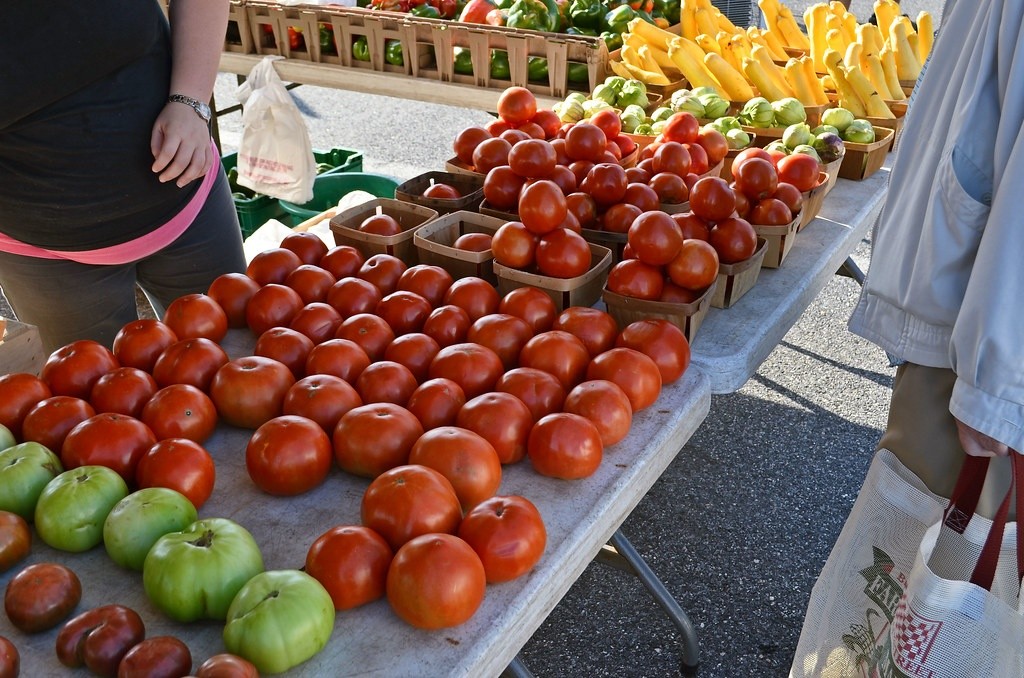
[280,172,401,228]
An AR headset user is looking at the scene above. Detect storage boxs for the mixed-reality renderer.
[220,146,364,242]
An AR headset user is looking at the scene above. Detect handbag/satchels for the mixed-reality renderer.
[789,445,1024,678]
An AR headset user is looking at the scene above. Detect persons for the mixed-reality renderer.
[846,0,1024,678]
[0,1,248,359]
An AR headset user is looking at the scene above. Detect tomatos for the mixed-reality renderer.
[360,84,820,303]
[0,230,690,678]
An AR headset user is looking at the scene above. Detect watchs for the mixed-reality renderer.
[168,94,211,124]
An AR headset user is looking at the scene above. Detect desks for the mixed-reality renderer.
[0,55,898,678]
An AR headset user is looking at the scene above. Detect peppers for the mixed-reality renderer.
[262,0,681,85]
